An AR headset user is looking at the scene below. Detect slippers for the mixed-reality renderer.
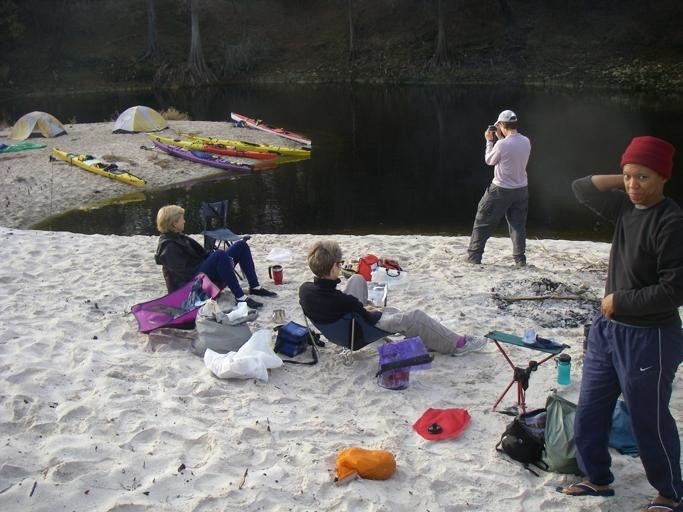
[556,481,616,496]
[639,496,683,512]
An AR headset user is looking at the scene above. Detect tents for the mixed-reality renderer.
[111,106,169,134]
[9,111,68,141]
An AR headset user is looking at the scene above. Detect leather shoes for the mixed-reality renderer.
[250,287,276,297]
[236,297,263,308]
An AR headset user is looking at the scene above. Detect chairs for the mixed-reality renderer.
[297,298,396,365]
[203,199,241,252]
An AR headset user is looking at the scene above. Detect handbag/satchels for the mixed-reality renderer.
[191,300,258,355]
[378,257,401,276]
[608,398,640,457]
[359,255,378,281]
[544,394,612,473]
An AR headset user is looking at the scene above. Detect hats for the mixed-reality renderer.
[621,136,673,178]
[496,110,517,125]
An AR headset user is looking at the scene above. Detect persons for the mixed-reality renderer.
[299,240,487,355]
[154,205,278,308]
[556,136,683,511]
[463,110,535,270]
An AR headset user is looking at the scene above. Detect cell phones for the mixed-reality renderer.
[488,125,497,132]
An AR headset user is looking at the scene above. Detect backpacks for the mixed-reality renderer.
[495,409,549,477]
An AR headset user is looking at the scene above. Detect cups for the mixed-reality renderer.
[268,264,283,285]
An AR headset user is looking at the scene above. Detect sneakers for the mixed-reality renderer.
[461,254,481,264]
[514,256,526,266]
[454,335,488,357]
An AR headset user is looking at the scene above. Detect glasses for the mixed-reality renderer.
[336,260,345,265]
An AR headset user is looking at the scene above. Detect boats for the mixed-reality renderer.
[52,146,145,187]
[230,111,311,145]
[154,141,279,173]
[147,132,282,159]
[181,133,311,158]
